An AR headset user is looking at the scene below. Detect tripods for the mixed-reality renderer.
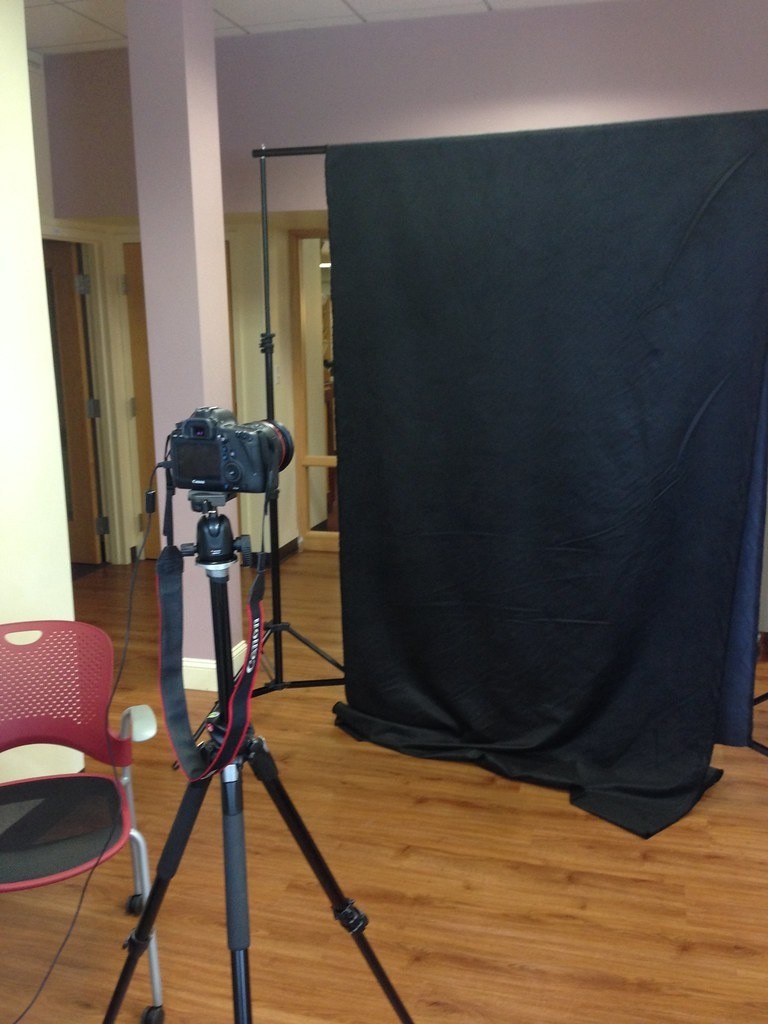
[102,490,413,1024]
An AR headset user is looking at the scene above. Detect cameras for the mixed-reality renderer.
[171,405,294,494]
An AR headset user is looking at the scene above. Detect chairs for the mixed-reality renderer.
[0,620,164,1024]
